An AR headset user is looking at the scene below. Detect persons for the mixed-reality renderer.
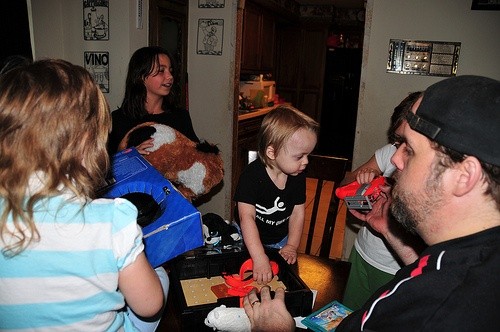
[243,74,499,332]
[338,91,426,309]
[0,59,170,332]
[105,46,225,208]
[233,105,320,285]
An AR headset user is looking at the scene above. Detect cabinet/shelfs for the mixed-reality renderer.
[238,103,291,185]
[242,8,276,73]
[273,18,331,125]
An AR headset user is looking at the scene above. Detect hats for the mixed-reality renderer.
[404,75,500,167]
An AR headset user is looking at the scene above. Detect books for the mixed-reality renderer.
[301,300,356,332]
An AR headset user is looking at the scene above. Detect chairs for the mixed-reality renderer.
[296,153,348,262]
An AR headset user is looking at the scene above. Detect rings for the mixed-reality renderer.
[251,300,260,308]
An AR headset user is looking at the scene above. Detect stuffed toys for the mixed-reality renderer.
[117,121,224,204]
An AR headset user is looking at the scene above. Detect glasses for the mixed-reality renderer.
[391,134,406,144]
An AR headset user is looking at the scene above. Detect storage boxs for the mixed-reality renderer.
[171,248,314,327]
